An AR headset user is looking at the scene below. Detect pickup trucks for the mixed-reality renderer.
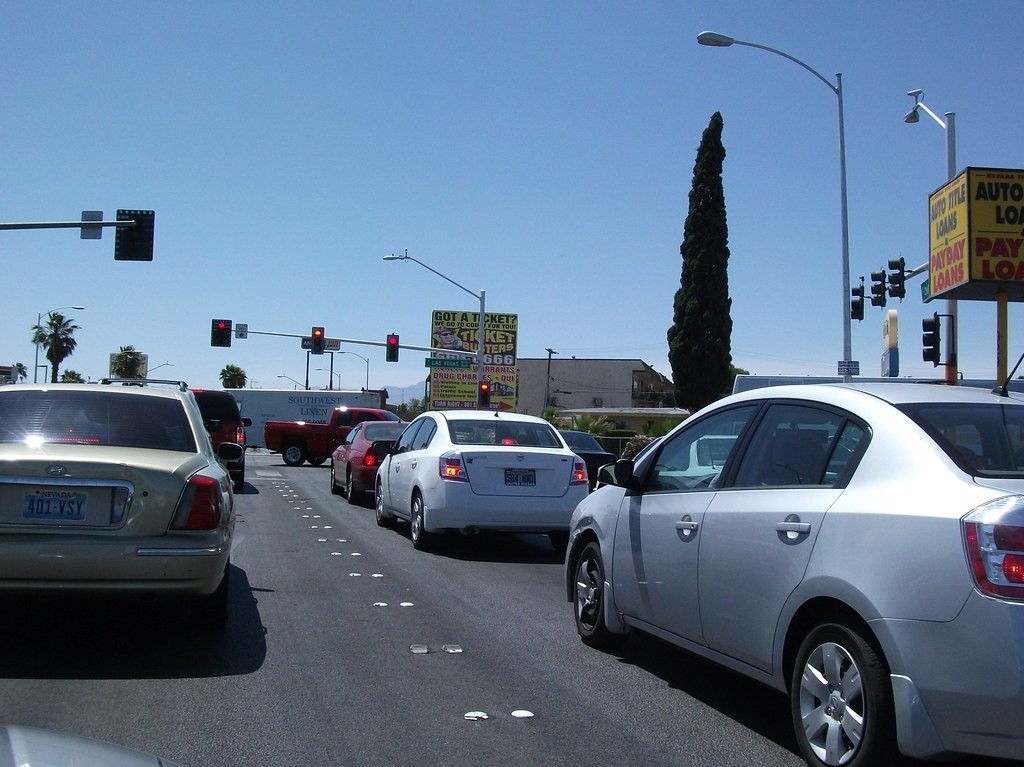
[264,408,404,467]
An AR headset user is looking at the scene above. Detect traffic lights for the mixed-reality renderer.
[386,333,399,363]
[871,269,886,308]
[851,276,864,320]
[311,327,324,355]
[887,257,906,299]
[211,319,232,348]
[921,312,940,368]
[478,381,491,408]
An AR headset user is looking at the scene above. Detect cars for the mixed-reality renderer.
[564,380,1024,767]
[371,410,590,554]
[536,427,618,496]
[595,434,741,490]
[191,387,252,493]
[330,420,412,506]
[0,380,244,633]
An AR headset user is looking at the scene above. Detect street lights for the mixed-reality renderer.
[315,368,341,391]
[34,305,85,384]
[383,247,486,410]
[37,365,48,384]
[338,351,370,391]
[696,31,852,449]
[902,88,957,444]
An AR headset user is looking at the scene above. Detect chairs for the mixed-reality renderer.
[770,435,825,488]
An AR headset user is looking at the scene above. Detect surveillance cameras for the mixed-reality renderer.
[908,89,922,96]
